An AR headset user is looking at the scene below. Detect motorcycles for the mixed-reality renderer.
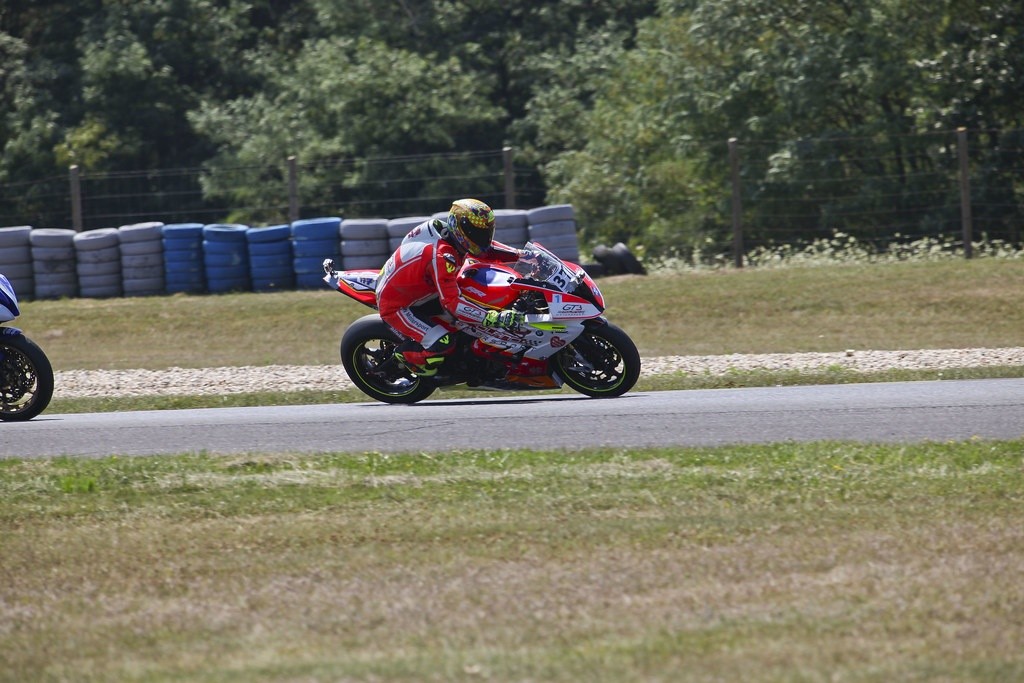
[322,240,641,409]
[0,275,55,421]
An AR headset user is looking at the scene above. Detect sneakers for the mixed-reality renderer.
[392,347,438,377]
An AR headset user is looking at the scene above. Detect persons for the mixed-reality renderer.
[375,198,548,377]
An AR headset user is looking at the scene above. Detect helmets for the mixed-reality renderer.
[448,199,496,256]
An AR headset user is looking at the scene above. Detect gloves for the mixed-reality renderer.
[483,311,525,332]
[519,248,544,266]
